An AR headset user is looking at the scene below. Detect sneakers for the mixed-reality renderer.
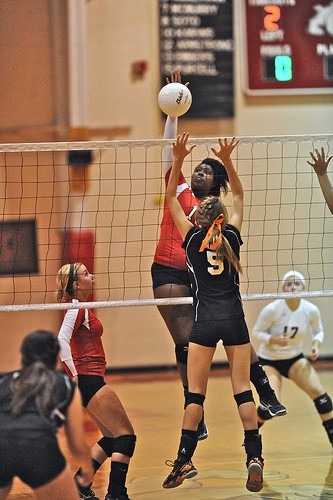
[260,393,288,417]
[162,460,198,488]
[105,493,130,500]
[197,425,208,440]
[74,474,99,500]
[246,457,263,492]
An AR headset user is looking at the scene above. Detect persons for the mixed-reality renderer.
[246,272,333,449]
[161,131,264,492]
[55,265,136,500]
[152,113,287,440]
[1,327,95,500]
[306,148,333,215]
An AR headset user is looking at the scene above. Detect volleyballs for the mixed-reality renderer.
[157,82,193,117]
